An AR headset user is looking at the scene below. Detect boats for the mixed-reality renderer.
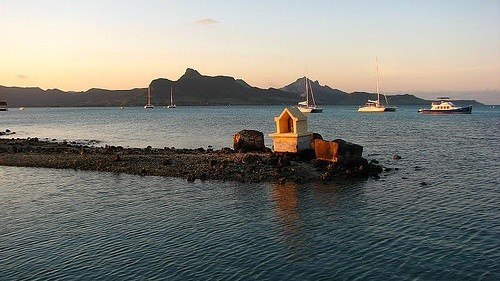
[418,97,475,115]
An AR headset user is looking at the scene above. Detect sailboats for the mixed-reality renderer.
[144,85,154,108]
[358,55,396,112]
[167,87,176,109]
[297,65,323,113]
